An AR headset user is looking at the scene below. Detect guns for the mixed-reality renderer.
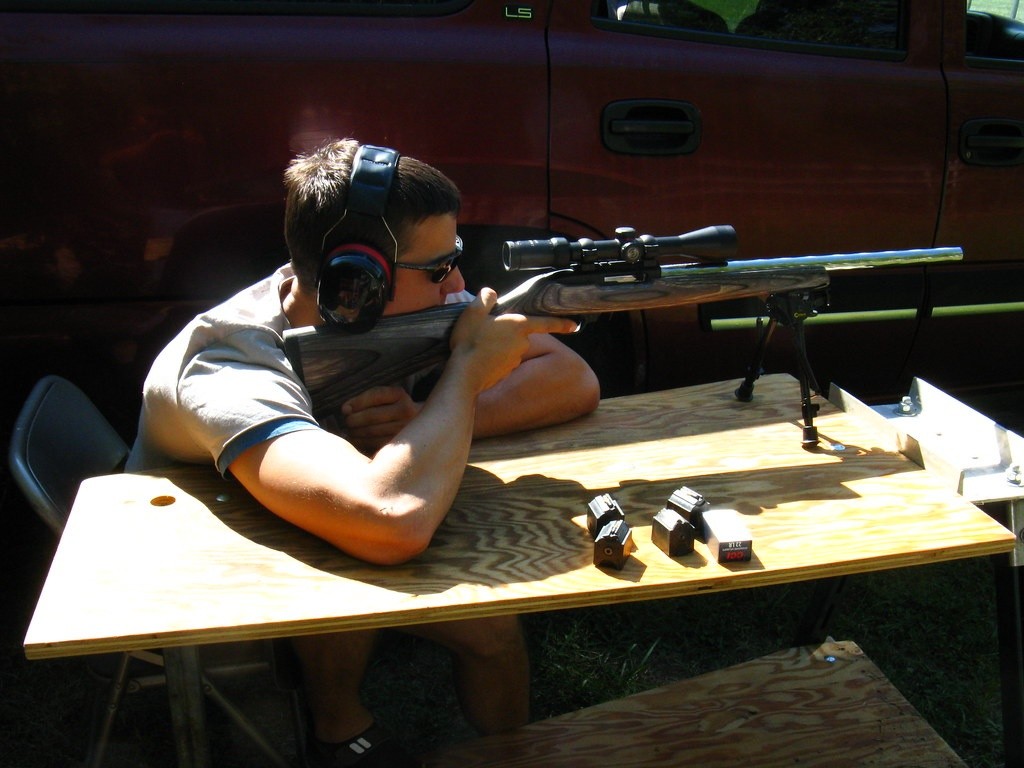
[284,224,964,453]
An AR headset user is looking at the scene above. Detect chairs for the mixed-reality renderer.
[8,373,311,768]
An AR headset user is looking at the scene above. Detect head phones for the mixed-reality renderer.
[314,146,400,337]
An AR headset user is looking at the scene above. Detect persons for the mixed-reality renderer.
[124,138,599,767]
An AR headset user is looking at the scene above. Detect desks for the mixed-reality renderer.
[22,373,1024,768]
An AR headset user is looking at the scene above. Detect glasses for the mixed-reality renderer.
[397,235,464,283]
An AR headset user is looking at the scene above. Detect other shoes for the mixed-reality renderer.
[306,723,423,768]
